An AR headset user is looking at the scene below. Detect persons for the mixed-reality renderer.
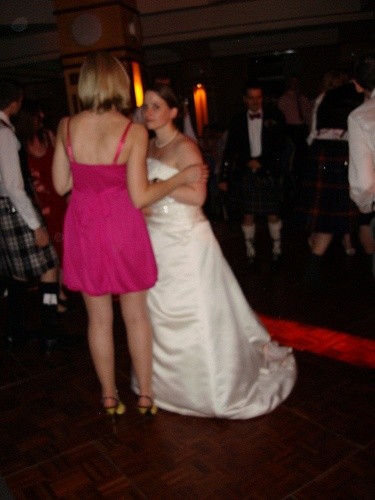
[131,80,298,420]
[52,50,209,430]
[0,84,68,320]
[179,53,375,289]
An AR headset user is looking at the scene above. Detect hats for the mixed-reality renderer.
[47,104,67,131]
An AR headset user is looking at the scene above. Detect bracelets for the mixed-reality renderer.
[152,177,158,185]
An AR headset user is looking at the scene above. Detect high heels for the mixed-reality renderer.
[342,239,356,256]
[136,396,158,422]
[101,397,126,420]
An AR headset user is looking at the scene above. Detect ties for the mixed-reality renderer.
[250,113,260,120]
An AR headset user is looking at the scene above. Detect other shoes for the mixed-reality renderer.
[270,251,284,270]
[4,335,28,351]
[41,336,64,352]
[243,252,260,270]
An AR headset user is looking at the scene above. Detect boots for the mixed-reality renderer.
[301,253,332,290]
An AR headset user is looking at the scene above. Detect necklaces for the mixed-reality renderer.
[152,127,178,147]
